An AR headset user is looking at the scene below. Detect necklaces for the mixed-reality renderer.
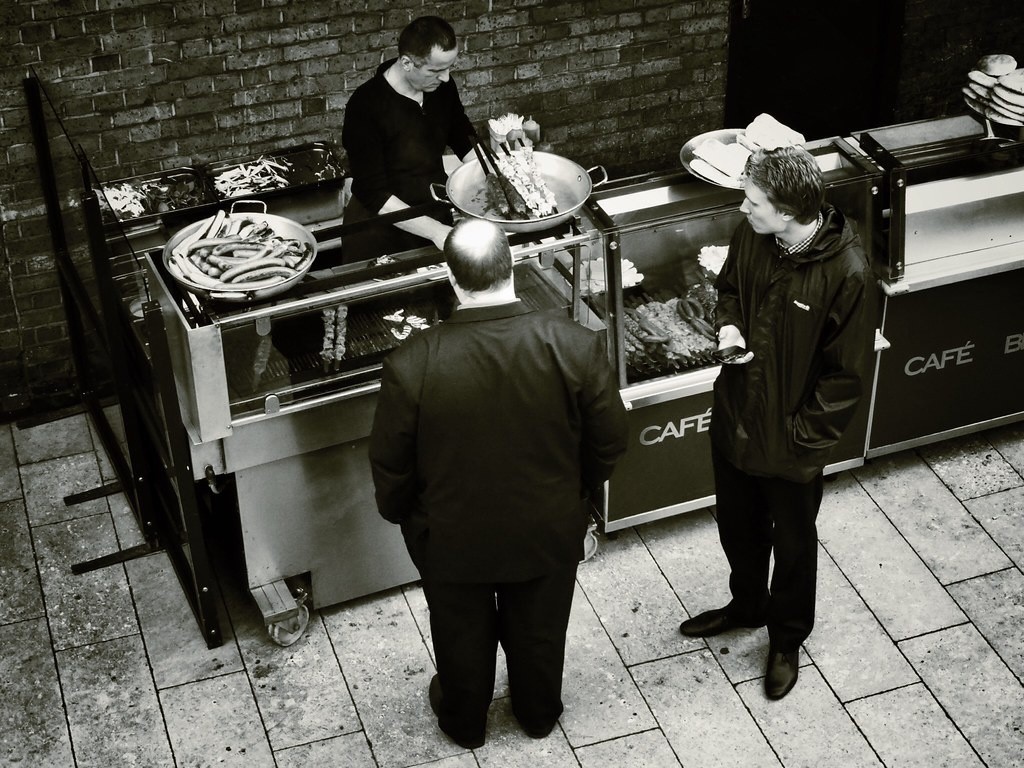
[386,67,391,84]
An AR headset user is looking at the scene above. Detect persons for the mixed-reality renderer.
[368,217,631,751]
[681,146,881,700]
[271,293,383,558]
[340,15,485,267]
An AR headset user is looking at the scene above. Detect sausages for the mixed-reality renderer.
[624,306,670,343]
[168,208,311,289]
[676,296,718,341]
[486,171,525,215]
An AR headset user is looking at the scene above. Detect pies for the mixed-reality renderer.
[961,52,1024,126]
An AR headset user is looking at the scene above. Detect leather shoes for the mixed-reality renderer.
[429,674,484,749]
[765,639,798,700]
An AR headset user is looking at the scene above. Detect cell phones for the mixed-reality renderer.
[711,346,747,361]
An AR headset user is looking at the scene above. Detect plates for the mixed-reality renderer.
[680,128,748,190]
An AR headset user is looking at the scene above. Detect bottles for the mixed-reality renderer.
[506,115,555,154]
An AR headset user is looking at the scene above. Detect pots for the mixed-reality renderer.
[429,150,608,232]
[162,200,318,302]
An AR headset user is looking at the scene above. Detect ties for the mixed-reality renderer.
[680,599,766,637]
